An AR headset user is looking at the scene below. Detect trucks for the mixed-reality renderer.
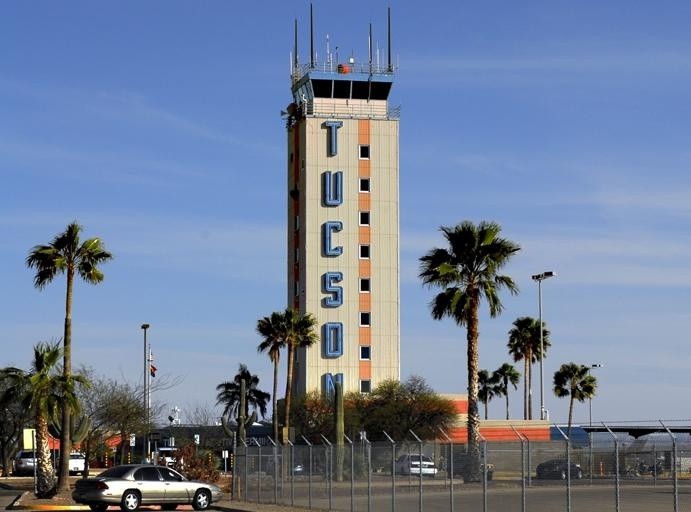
[157,447,183,471]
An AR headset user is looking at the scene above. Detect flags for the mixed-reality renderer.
[147,347,158,379]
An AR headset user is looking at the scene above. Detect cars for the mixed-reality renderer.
[72,464,225,512]
[392,452,437,477]
[10,450,90,476]
[536,460,583,481]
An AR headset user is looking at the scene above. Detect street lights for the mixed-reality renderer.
[141,323,150,463]
[530,271,555,420]
[581,363,604,483]
